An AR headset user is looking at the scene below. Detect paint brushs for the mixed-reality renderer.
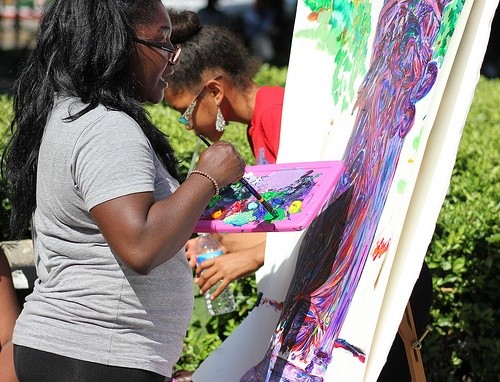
[196,133,280,219]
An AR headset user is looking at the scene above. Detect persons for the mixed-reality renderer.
[0,0,247,382]
[0,246,23,382]
[162,9,285,382]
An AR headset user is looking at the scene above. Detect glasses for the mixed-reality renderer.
[178,73,226,125]
[133,37,182,63]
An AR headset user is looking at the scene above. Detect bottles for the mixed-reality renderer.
[194,232,235,316]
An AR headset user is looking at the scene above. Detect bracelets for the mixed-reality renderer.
[190,170,220,198]
